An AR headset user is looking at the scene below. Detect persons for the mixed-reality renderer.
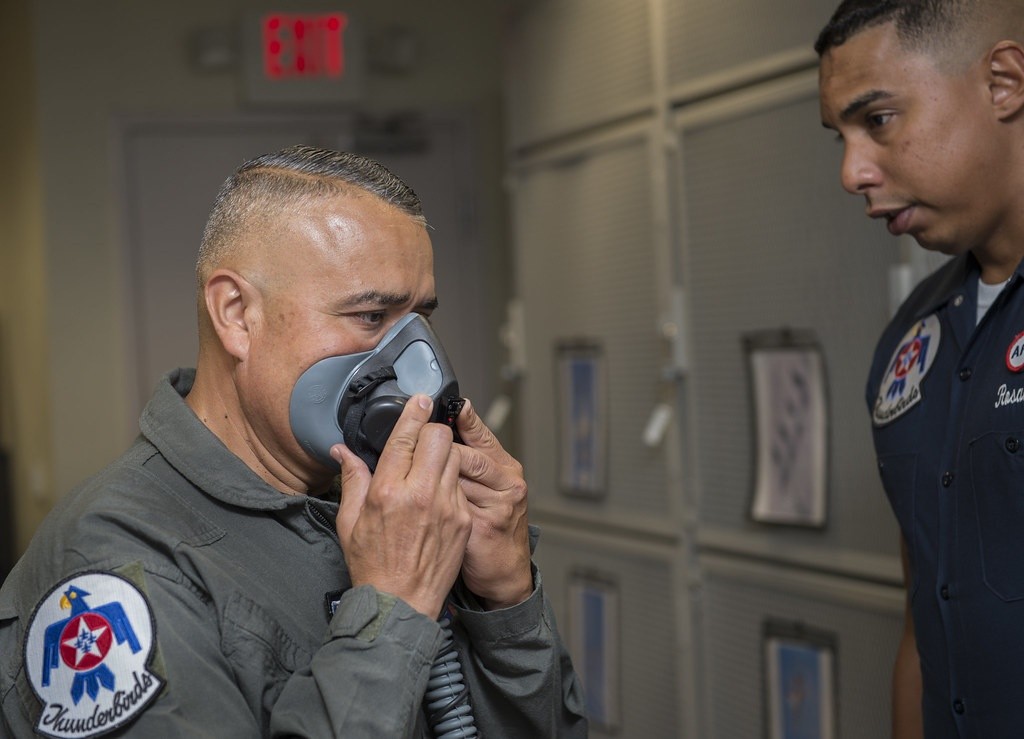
[0,145,589,739]
[813,0,1024,739]
